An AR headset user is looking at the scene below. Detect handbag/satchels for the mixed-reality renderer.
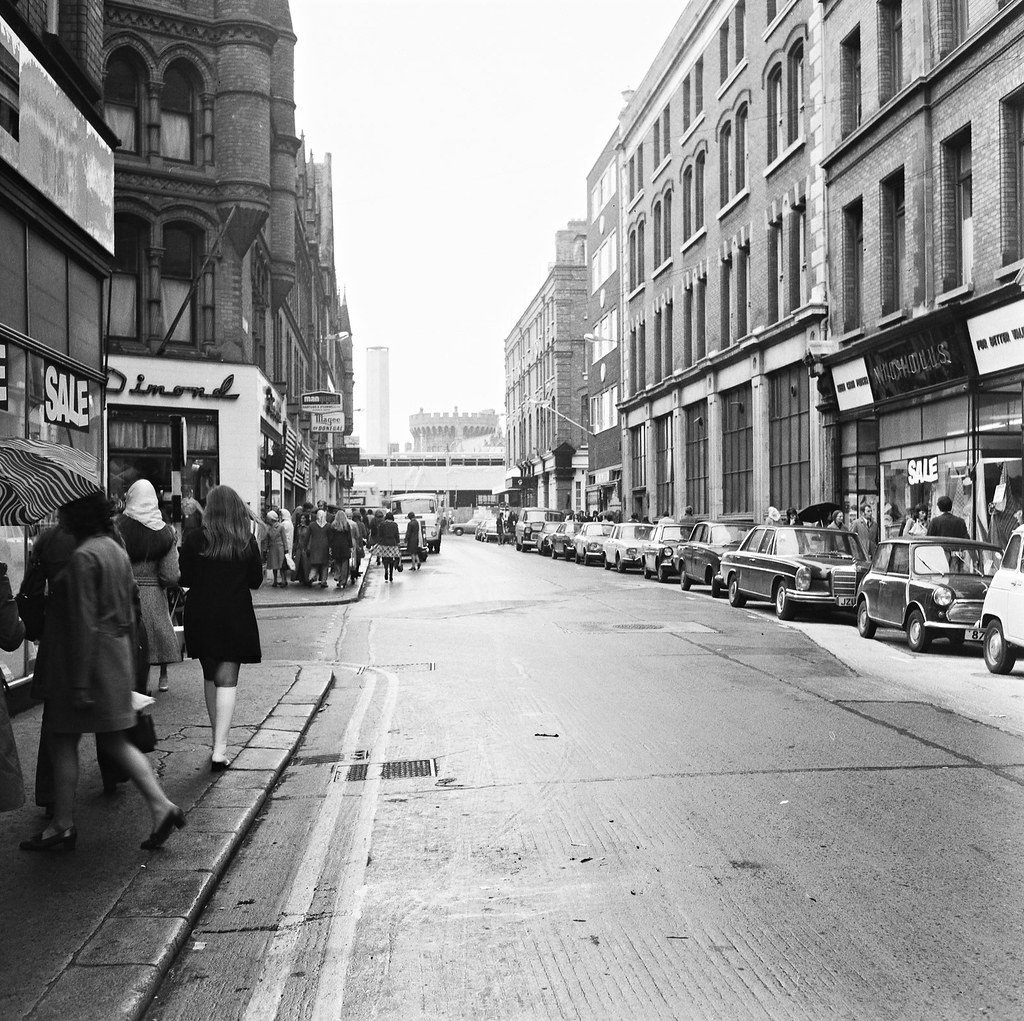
[131,711,158,754]
[395,556,402,572]
[13,527,60,642]
[284,551,296,572]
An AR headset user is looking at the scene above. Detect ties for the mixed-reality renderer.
[866,522,870,529]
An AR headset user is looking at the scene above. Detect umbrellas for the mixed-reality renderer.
[797,501,840,527]
[0,436,100,526]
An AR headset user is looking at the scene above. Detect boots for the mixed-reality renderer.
[202,678,237,771]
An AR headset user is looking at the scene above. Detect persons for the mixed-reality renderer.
[441,517,454,529]
[565,510,614,523]
[0,562,26,811]
[369,510,384,565]
[628,511,674,523]
[926,496,978,573]
[369,512,400,583]
[404,512,423,571]
[496,511,518,546]
[264,500,374,589]
[850,505,879,560]
[827,510,848,551]
[19,485,188,857]
[680,506,699,536]
[178,485,264,772]
[766,506,810,554]
[17,479,203,820]
[898,503,928,537]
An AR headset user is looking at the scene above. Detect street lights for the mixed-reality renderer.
[583,332,621,412]
[315,331,348,390]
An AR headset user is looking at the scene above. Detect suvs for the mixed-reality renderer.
[514,507,564,553]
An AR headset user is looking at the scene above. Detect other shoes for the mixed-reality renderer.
[44,802,53,818]
[272,579,357,589]
[158,677,168,692]
[416,563,421,571]
[384,573,388,580]
[391,577,393,581]
[409,566,415,570]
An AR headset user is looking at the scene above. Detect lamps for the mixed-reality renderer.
[583,334,622,343]
[314,332,351,344]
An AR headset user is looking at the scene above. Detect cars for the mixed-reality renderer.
[853,536,1004,652]
[977,523,1024,676]
[673,522,760,600]
[720,526,872,621]
[537,520,695,584]
[386,493,443,554]
[475,518,510,544]
[449,519,481,536]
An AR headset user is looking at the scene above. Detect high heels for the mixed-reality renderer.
[142,807,187,850]
[19,824,76,851]
